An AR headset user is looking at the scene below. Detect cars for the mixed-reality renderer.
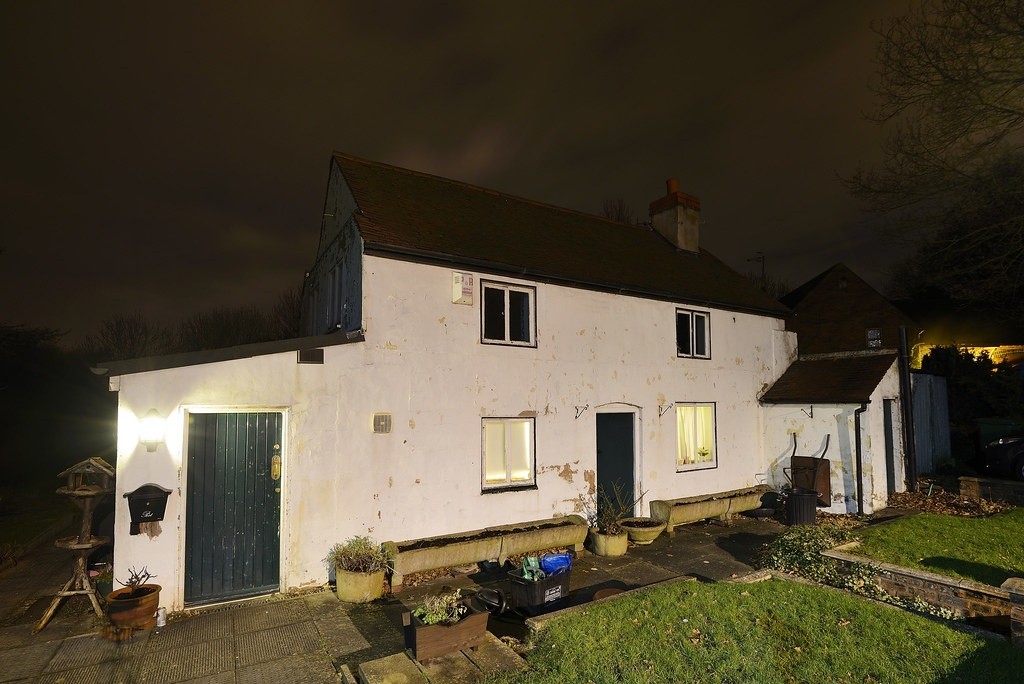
[980,429,1024,480]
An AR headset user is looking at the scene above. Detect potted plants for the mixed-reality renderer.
[322,535,395,604]
[105,566,162,629]
[402,592,491,666]
[580,481,649,556]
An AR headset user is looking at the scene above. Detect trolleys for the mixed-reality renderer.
[784,431,833,507]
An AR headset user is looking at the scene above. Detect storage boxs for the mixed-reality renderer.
[507,562,570,616]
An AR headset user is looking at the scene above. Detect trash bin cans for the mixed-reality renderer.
[506,559,572,618]
[785,488,818,523]
